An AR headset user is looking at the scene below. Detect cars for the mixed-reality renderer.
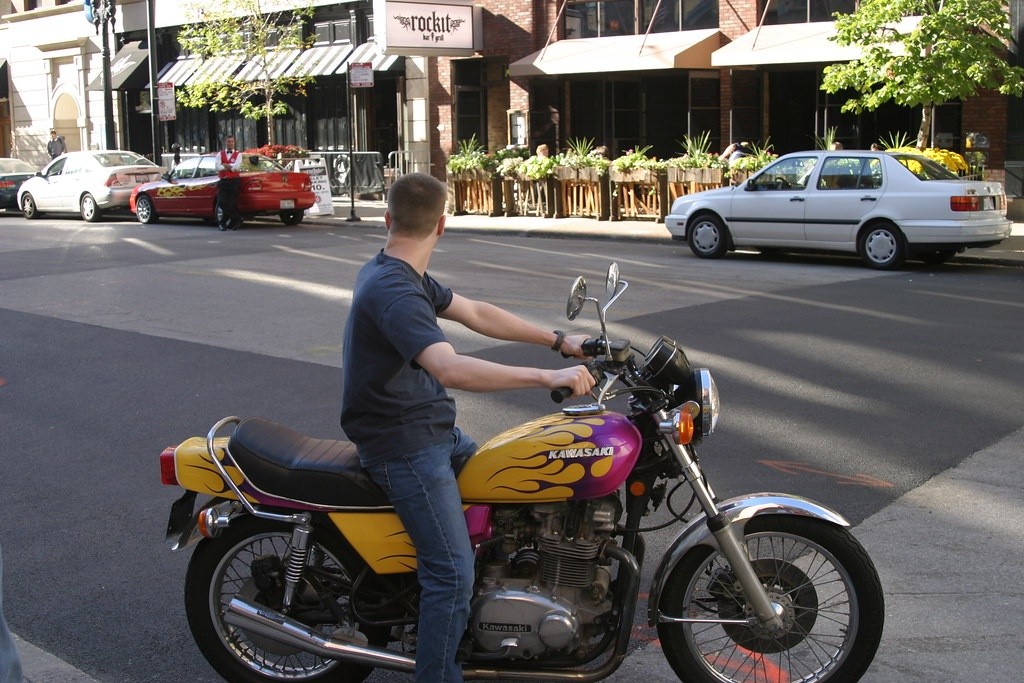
[17,150,167,222]
[0,158,38,208]
[665,150,1014,269]
[130,154,315,229]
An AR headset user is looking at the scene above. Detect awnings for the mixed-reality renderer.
[85,41,150,92]
[281,44,353,78]
[185,55,247,86]
[335,43,399,74]
[710,16,951,67]
[509,29,719,77]
[145,59,204,88]
[231,49,300,82]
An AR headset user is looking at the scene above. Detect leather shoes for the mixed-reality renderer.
[219,218,226,231]
[233,216,244,231]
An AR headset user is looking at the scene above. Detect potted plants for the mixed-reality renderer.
[452,137,773,184]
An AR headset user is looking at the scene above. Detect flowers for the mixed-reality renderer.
[884,146,969,181]
[243,143,309,168]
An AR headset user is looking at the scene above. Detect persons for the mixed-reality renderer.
[47,131,67,176]
[215,136,245,230]
[729,143,748,172]
[869,144,879,173]
[536,144,549,158]
[340,173,595,683]
[820,142,843,189]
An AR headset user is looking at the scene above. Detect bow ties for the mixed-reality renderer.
[227,151,232,153]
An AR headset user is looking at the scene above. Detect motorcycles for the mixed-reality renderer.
[160,262,885,683]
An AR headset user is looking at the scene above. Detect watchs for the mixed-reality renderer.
[551,330,566,352]
